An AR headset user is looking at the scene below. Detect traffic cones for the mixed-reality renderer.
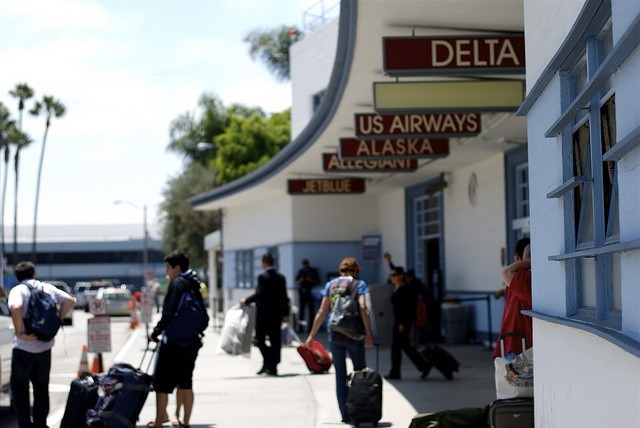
[78,345,90,379]
[91,352,105,373]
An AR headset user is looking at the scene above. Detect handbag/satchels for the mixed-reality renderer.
[409,403,491,427]
[414,299,427,327]
[328,292,367,341]
[494,349,533,399]
[220,300,256,354]
[87,334,160,428]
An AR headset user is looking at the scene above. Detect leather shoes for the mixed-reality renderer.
[257,361,270,373]
[266,366,278,376]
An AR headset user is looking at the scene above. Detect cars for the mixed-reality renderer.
[85,281,115,312]
[73,281,90,309]
[94,287,136,316]
[40,281,76,326]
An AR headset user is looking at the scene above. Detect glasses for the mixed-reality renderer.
[390,271,399,274]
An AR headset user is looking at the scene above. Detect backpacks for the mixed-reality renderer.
[164,276,204,346]
[20,280,63,341]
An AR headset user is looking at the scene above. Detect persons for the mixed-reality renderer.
[6,259,78,428]
[239,250,291,377]
[491,236,534,358]
[147,247,211,427]
[295,258,322,334]
[302,256,376,422]
[380,251,445,381]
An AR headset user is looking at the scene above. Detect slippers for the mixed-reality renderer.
[173,421,190,428]
[146,420,171,427]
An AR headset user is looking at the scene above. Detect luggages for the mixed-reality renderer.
[285,320,332,373]
[281,321,292,347]
[61,371,98,428]
[344,340,382,428]
[421,327,460,378]
[486,332,534,427]
